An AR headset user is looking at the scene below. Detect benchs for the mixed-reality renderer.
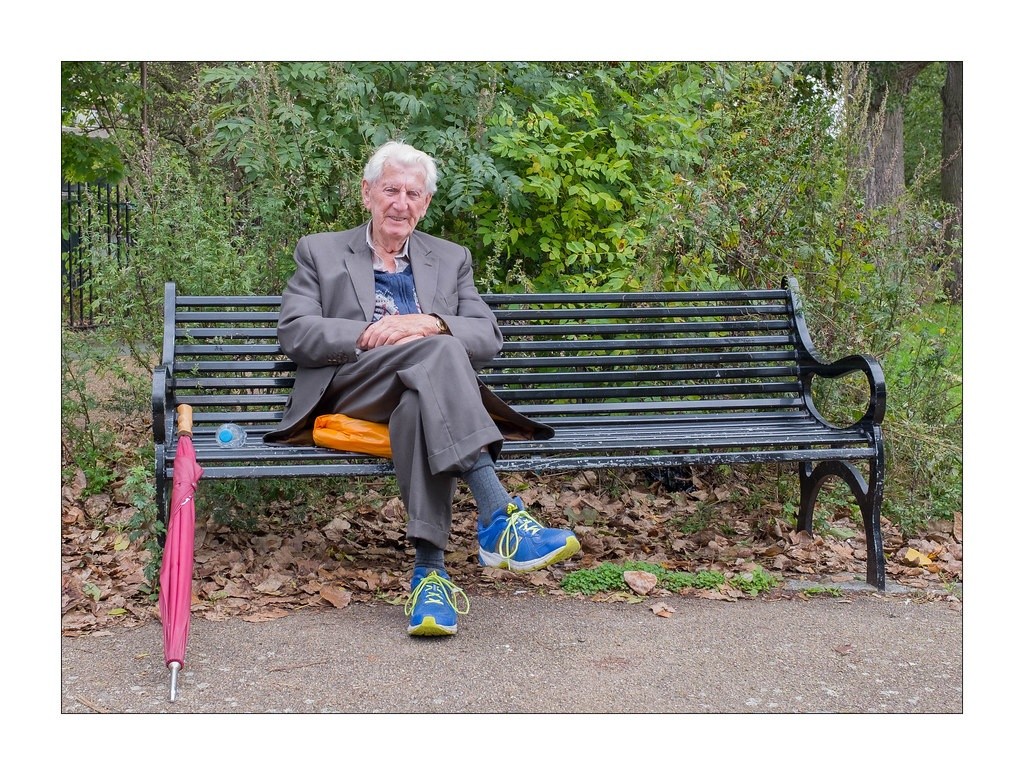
[149,282,886,592]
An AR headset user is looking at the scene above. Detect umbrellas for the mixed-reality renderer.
[158,404,204,702]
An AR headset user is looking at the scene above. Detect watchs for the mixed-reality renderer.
[434,316,449,334]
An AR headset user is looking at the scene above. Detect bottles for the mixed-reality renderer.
[215,424,248,449]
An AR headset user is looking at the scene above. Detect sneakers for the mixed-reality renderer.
[404,567,470,637]
[477,496,582,573]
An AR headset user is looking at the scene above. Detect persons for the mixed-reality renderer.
[278,142,583,637]
